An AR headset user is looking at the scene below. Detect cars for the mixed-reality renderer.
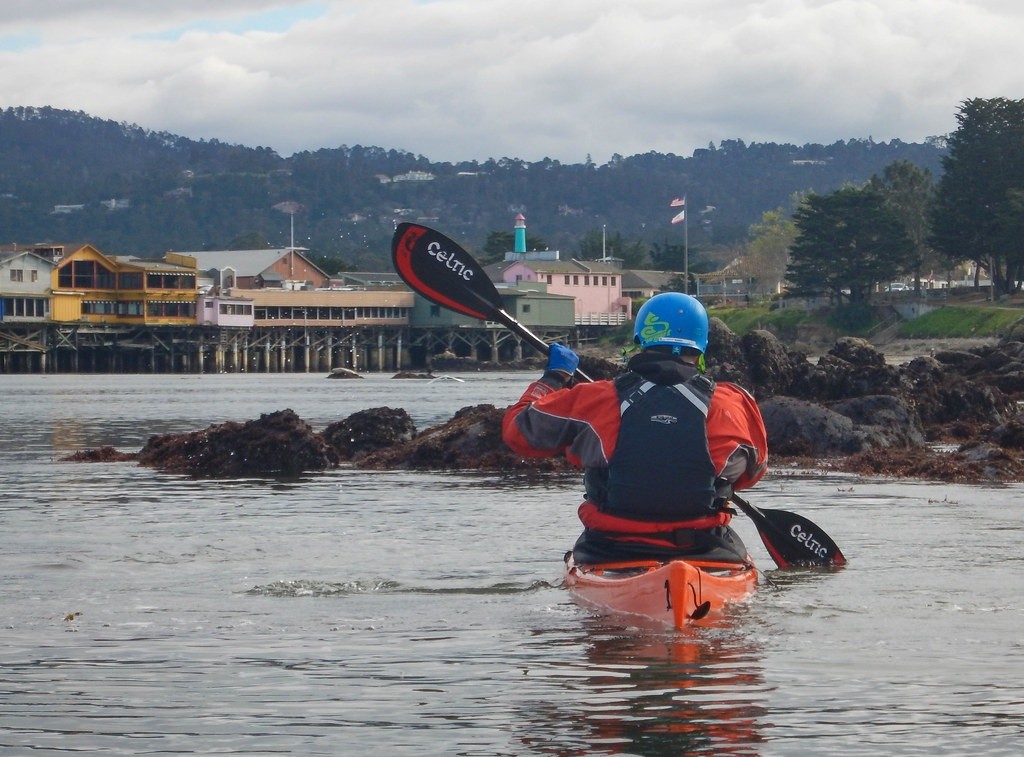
[882,282,910,291]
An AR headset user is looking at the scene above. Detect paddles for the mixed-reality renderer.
[389,222,849,569]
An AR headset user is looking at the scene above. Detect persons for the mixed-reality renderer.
[502,292,768,565]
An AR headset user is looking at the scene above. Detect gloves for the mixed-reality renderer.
[547,342,579,386]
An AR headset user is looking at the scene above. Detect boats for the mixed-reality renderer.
[563,550,761,632]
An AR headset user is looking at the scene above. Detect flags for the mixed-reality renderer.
[672,209,687,224]
[670,192,686,207]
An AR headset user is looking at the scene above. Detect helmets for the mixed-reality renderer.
[633,292,708,360]
